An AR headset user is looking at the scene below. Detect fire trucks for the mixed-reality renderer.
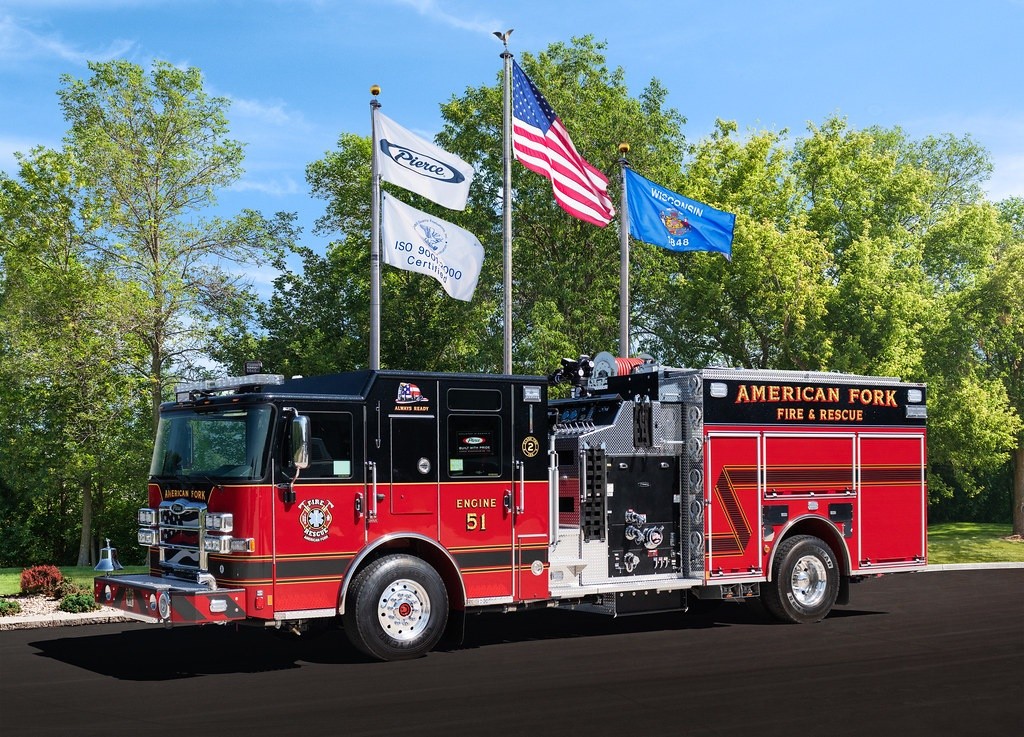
[91,349,930,664]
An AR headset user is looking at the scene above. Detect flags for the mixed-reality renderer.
[382,190,484,302]
[511,58,615,227]
[373,110,474,211]
[625,167,736,262]
